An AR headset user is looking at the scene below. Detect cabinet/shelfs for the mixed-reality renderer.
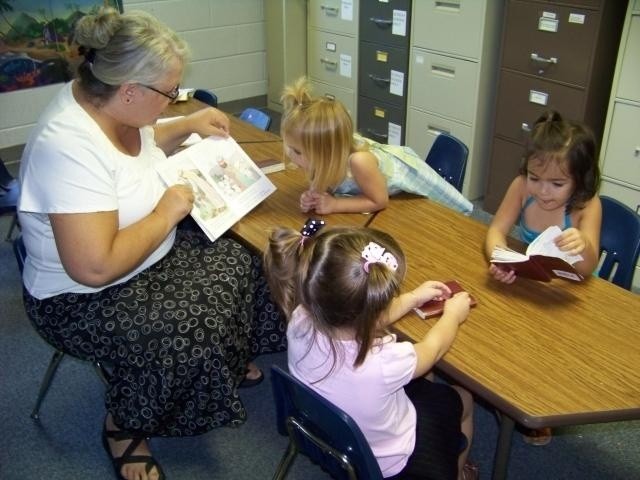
[593,2,639,292]
[260,0,629,232]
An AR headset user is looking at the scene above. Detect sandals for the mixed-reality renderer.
[101,411,166,480]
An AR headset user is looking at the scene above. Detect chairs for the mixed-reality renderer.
[1,155,23,240]
[265,362,387,479]
[6,231,123,423]
[193,87,218,110]
[238,105,273,132]
[593,193,640,293]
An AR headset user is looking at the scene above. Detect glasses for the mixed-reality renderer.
[148,85,180,100]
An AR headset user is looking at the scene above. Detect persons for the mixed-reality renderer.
[16,8,287,480]
[485,113,604,450]
[280,75,473,218]
[267,217,484,477]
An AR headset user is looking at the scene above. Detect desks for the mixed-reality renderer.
[106,88,638,479]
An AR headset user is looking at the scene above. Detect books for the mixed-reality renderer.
[160,115,203,146]
[489,227,583,286]
[412,282,474,321]
[154,127,274,246]
